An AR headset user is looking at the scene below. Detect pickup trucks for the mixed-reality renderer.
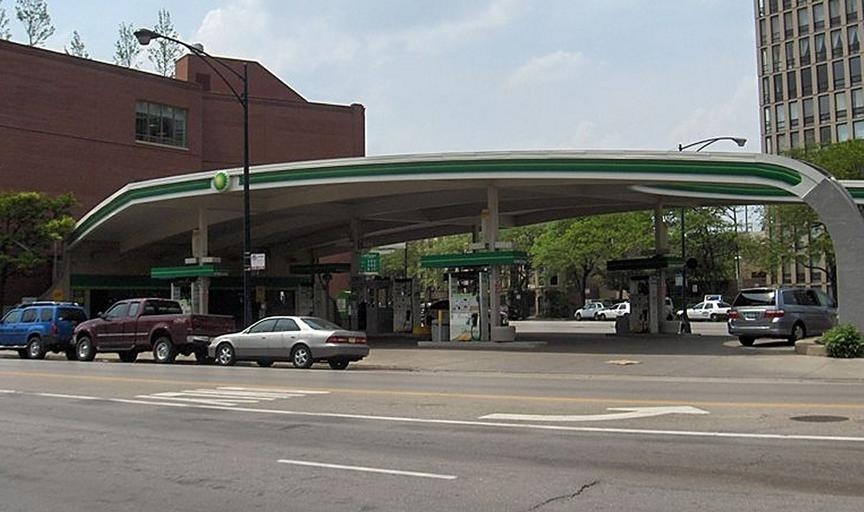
[66,297,237,363]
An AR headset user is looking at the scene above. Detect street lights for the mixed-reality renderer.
[132,25,255,315]
[679,134,750,334]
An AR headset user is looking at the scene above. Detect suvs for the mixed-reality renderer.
[0,299,87,360]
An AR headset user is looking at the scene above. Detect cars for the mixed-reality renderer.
[423,300,509,329]
[675,299,731,322]
[202,312,372,371]
[573,299,613,322]
[597,300,630,320]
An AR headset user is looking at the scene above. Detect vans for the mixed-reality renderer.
[725,284,837,346]
[664,297,675,314]
